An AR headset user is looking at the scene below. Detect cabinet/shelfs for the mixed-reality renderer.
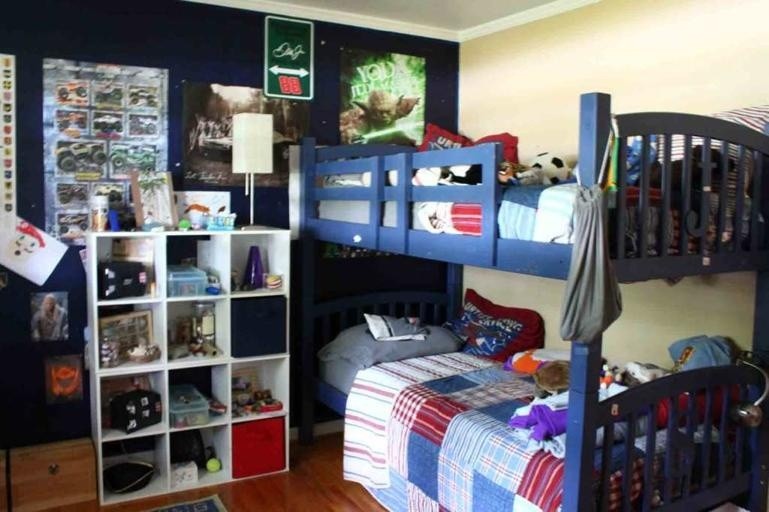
[86,230,291,505]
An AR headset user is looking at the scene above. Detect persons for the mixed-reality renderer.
[30,293,68,341]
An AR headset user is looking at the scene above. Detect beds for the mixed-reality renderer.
[296,92,768,509]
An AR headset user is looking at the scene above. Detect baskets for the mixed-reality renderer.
[200,211,237,230]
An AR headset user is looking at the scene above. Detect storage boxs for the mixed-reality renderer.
[167,263,207,297]
[168,384,211,428]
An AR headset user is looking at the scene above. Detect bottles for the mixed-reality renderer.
[191,302,215,346]
[88,195,109,231]
[243,244,263,289]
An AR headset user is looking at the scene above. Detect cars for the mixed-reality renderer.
[198,121,294,168]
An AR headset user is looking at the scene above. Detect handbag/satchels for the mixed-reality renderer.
[98,260,147,299]
[108,388,162,434]
[558,129,622,346]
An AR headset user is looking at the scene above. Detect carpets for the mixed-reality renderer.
[149,492,229,512]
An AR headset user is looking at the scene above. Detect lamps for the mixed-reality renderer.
[231,112,274,229]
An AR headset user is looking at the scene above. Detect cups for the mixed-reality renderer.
[261,271,283,290]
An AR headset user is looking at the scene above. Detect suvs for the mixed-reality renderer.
[49,75,161,239]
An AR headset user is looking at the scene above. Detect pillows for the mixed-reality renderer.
[653,101,769,200]
[315,288,544,370]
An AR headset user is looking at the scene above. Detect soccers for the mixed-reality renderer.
[531,153,569,187]
